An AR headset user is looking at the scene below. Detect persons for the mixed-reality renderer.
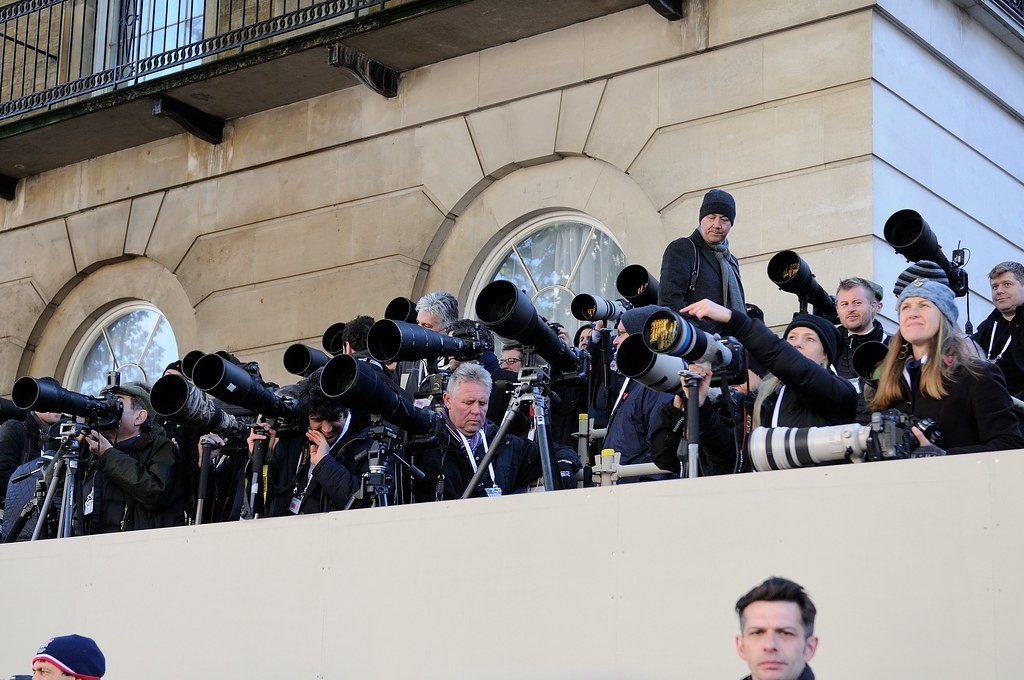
[865,277,1024,458]
[405,360,525,505]
[0,189,1024,543]
[735,576,818,680]
[31,634,105,680]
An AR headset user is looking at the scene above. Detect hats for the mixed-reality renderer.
[895,278,959,328]
[699,189,736,227]
[865,279,883,302]
[621,305,670,335]
[893,261,949,295]
[32,635,106,680]
[783,314,842,364]
[100,384,151,412]
[163,360,184,376]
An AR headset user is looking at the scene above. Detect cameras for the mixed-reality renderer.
[320,354,445,448]
[569,265,747,427]
[883,209,968,297]
[851,340,890,392]
[475,280,592,387]
[11,371,124,432]
[366,319,481,363]
[766,250,842,325]
[748,408,916,472]
[149,296,420,456]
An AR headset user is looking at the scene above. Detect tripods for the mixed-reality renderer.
[4,422,91,544]
[459,343,564,500]
[343,427,426,511]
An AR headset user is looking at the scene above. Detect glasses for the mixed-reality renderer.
[618,329,626,337]
[500,357,521,366]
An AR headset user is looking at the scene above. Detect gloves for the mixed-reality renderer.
[558,461,575,489]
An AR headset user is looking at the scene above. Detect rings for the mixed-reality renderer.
[698,308,701,314]
[318,439,321,442]
